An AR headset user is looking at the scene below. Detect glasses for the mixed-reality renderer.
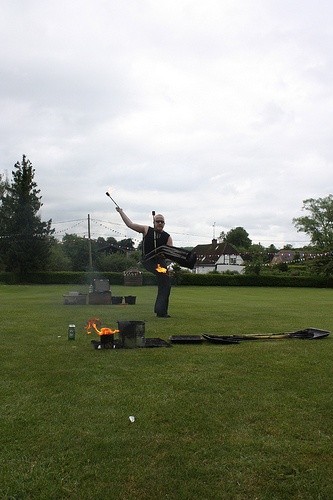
[156,221,164,223]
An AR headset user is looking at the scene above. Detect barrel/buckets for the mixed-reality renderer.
[112,296,136,304]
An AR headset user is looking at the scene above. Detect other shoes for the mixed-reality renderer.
[157,313,171,318]
[187,253,196,270]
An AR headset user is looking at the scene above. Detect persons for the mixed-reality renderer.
[116,206,198,318]
